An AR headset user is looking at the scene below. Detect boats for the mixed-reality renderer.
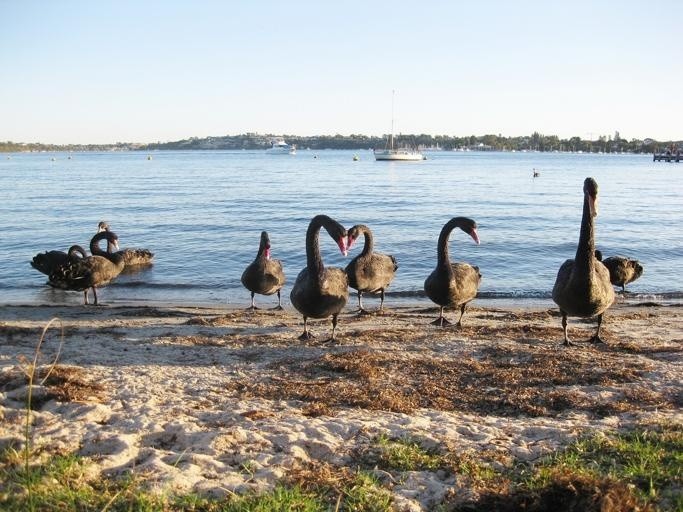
[451,144,470,151]
[263,141,296,154]
[373,89,425,160]
[418,142,442,151]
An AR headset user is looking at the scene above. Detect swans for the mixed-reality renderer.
[241,231,286,311]
[424,216,483,327]
[29,222,155,307]
[345,224,398,316]
[290,215,349,343]
[552,177,616,348]
[594,249,644,293]
